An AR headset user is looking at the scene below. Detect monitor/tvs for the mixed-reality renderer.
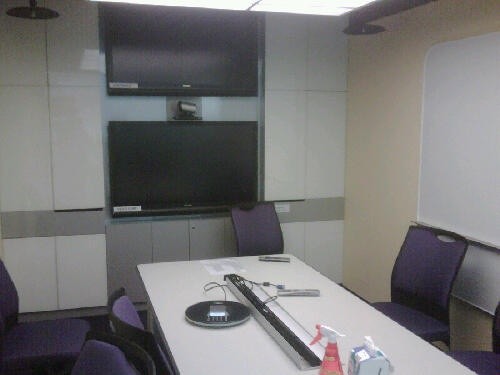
[108,120,257,217]
[103,2,258,96]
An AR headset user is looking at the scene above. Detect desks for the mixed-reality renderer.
[136,253,478,375]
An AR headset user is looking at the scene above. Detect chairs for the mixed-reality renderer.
[107,286,167,375]
[71,331,156,375]
[0,260,90,372]
[371,225,469,351]
[228,201,284,256]
[444,302,500,375]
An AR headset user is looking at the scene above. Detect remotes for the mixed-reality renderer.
[277,289,320,296]
[259,256,290,262]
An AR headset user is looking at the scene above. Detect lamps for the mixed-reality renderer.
[6,0,60,19]
[342,22,386,34]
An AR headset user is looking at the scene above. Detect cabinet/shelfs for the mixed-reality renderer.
[105,216,239,304]
